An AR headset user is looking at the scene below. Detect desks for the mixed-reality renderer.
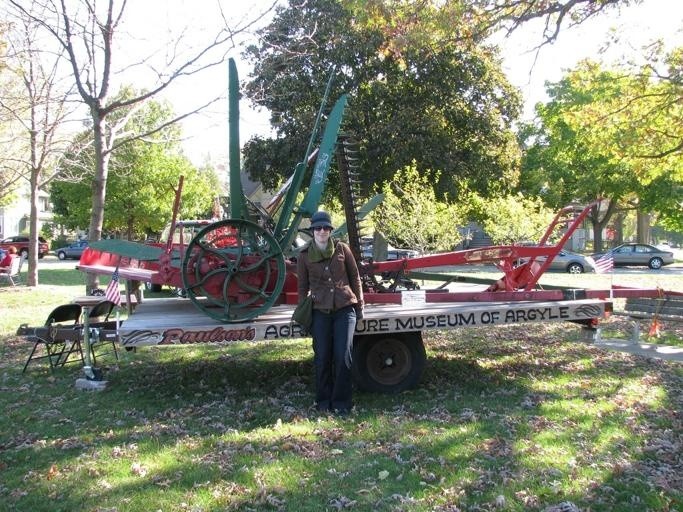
[74,294,137,354]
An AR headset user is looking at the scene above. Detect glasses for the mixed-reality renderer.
[314,227,330,231]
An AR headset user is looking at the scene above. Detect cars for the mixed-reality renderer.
[358,235,420,261]
[588,242,676,272]
[515,243,596,275]
[54,237,95,261]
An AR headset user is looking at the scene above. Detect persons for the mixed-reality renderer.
[296,211,365,416]
[0,245,17,273]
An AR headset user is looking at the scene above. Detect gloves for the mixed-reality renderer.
[356,308,364,320]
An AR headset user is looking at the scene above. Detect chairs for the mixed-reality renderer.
[0,251,28,287]
[16,300,118,375]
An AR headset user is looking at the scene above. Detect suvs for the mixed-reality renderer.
[0,233,49,263]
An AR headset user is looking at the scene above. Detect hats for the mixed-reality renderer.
[310,212,333,230]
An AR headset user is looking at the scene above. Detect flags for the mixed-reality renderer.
[104,256,121,308]
[593,249,613,274]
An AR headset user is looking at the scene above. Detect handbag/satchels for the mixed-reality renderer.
[291,294,313,330]
